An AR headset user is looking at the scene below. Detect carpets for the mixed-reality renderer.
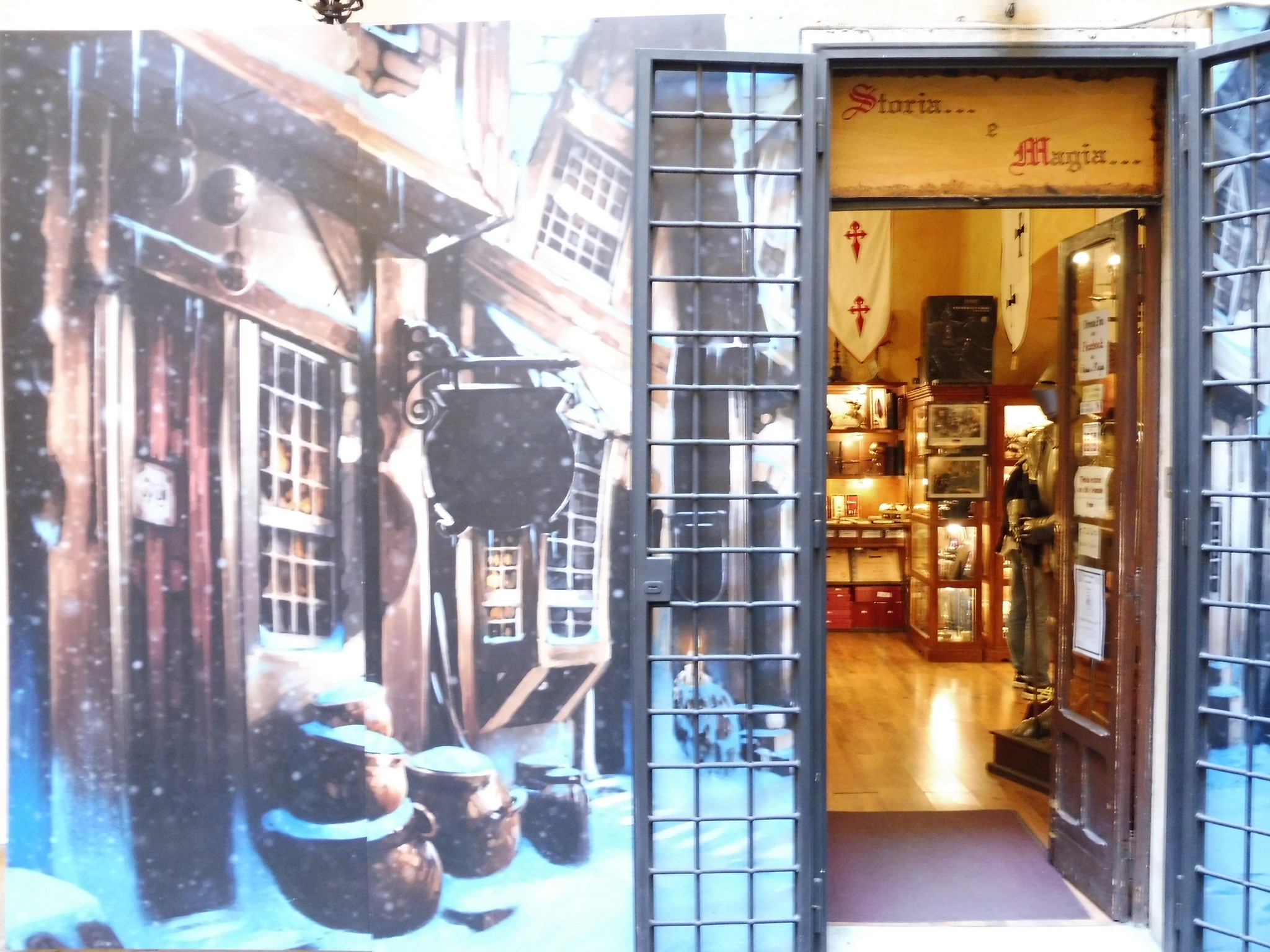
[826,807,1091,923]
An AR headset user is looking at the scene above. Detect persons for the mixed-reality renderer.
[994,457,1059,700]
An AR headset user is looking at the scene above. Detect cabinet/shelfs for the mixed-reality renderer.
[982,380,1054,661]
[904,382,984,665]
[825,379,906,633]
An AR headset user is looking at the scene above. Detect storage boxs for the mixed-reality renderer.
[826,586,904,633]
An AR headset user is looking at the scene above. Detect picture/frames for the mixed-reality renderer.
[923,452,990,502]
[866,386,890,432]
[924,399,992,450]
